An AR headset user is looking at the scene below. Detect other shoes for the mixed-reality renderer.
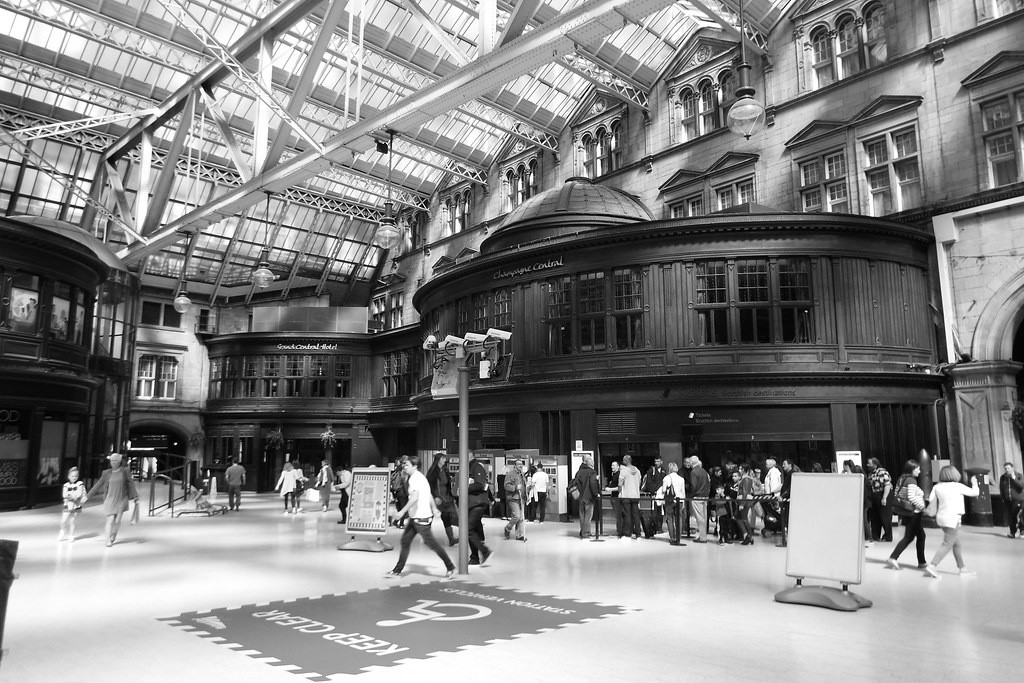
[59,529,66,540]
[917,562,928,568]
[445,568,454,579]
[504,528,510,539]
[337,520,345,524]
[888,558,899,569]
[693,539,707,543]
[1008,534,1014,538]
[959,567,976,575]
[516,537,527,540]
[282,507,302,514]
[384,570,401,578]
[1019,534,1024,539]
[468,559,480,565]
[68,535,74,541]
[106,537,112,547]
[480,550,493,566]
[926,563,941,579]
[110,532,117,542]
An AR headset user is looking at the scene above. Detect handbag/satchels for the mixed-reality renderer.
[566,471,582,500]
[641,466,655,492]
[892,476,915,517]
[664,474,676,499]
[130,503,139,525]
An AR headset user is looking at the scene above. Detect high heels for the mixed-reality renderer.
[741,535,754,545]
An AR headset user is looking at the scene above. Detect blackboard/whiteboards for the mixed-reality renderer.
[785,473,864,585]
[345,467,391,536]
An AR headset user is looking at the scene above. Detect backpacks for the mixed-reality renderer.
[389,470,404,493]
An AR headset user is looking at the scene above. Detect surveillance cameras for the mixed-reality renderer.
[486,328,512,341]
[444,335,468,346]
[423,335,436,349]
[438,341,453,348]
[465,332,490,342]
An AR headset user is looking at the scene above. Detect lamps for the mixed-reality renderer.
[252,191,275,289]
[725,0,766,141]
[172,230,195,314]
[376,129,400,249]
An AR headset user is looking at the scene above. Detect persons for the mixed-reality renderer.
[224,457,246,512]
[688,456,709,543]
[886,460,928,569]
[334,466,352,524]
[925,466,980,579]
[57,466,87,542]
[274,447,1024,583]
[661,462,686,542]
[319,459,334,512]
[385,456,459,583]
[86,453,140,547]
[575,454,600,538]
[618,455,642,540]
[503,459,530,540]
[732,463,755,543]
